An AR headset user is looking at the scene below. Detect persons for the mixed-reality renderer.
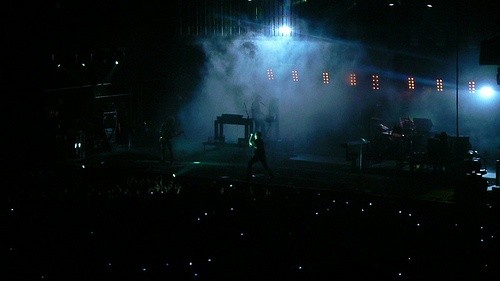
[269,96,280,135]
[249,96,262,132]
[248,130,269,178]
[160,117,175,165]
